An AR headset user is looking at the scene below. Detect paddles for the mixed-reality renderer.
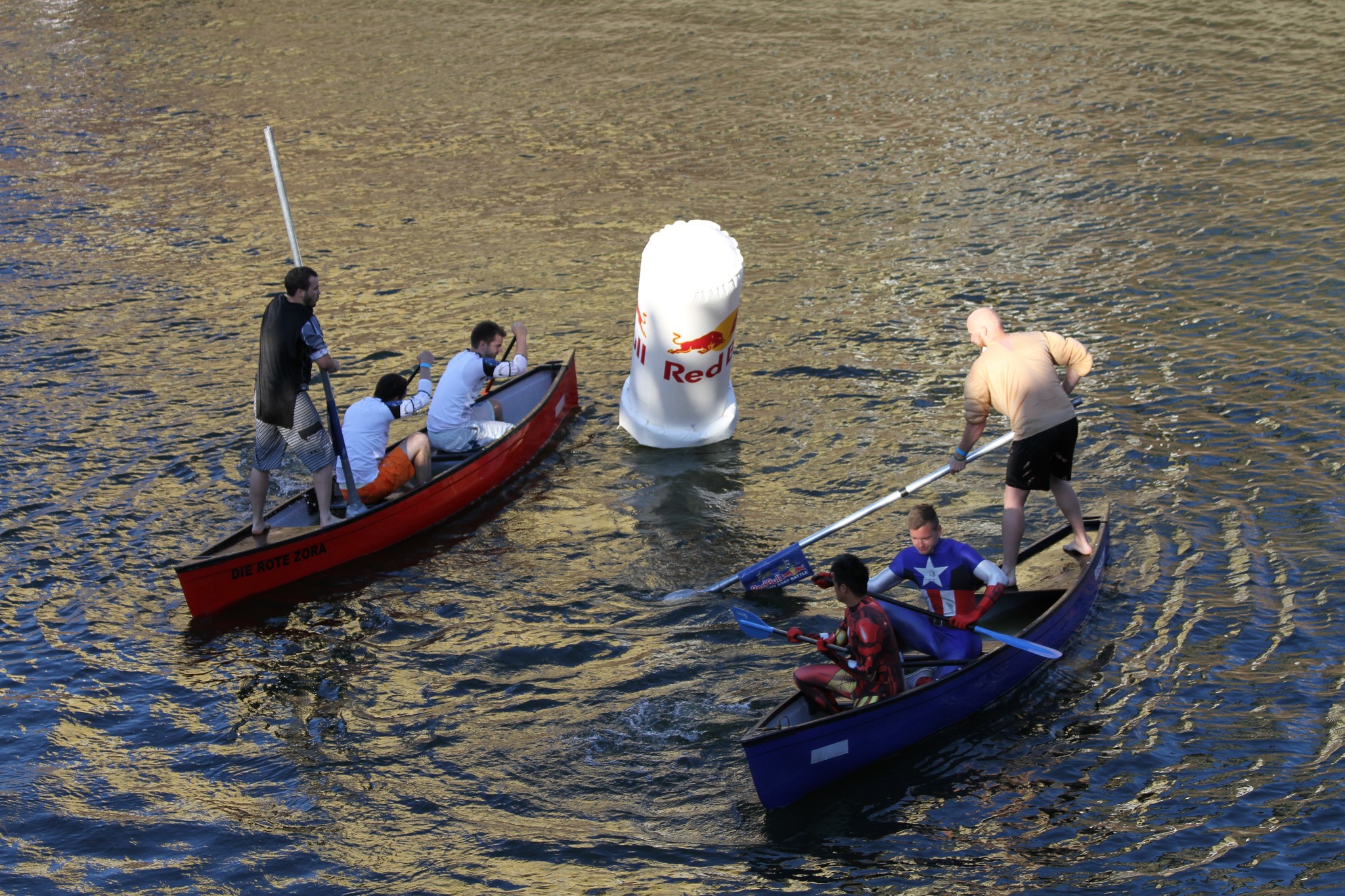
[730,606,852,655]
[824,573,1063,661]
[403,362,421,394]
[479,335,516,396]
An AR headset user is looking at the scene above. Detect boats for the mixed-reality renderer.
[173,344,578,619]
[741,498,1111,811]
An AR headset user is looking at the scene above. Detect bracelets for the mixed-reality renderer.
[954,454,967,461]
[420,363,432,370]
[956,448,968,457]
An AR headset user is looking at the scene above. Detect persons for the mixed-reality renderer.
[249,266,339,535]
[786,552,906,716]
[949,306,1094,588]
[425,321,528,454]
[336,350,434,506]
[811,504,1008,689]
